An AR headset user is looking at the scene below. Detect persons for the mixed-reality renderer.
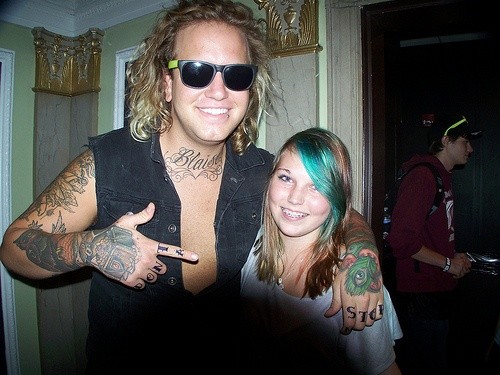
[386,103,485,375]
[0,0,386,375]
[238,126,404,375]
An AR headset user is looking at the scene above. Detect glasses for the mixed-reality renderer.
[443,115,470,140]
[166,59,259,92]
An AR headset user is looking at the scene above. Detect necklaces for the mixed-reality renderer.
[277,240,311,285]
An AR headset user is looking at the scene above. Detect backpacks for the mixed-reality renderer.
[381,160,444,256]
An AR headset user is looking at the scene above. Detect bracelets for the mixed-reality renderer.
[442,256,452,273]
